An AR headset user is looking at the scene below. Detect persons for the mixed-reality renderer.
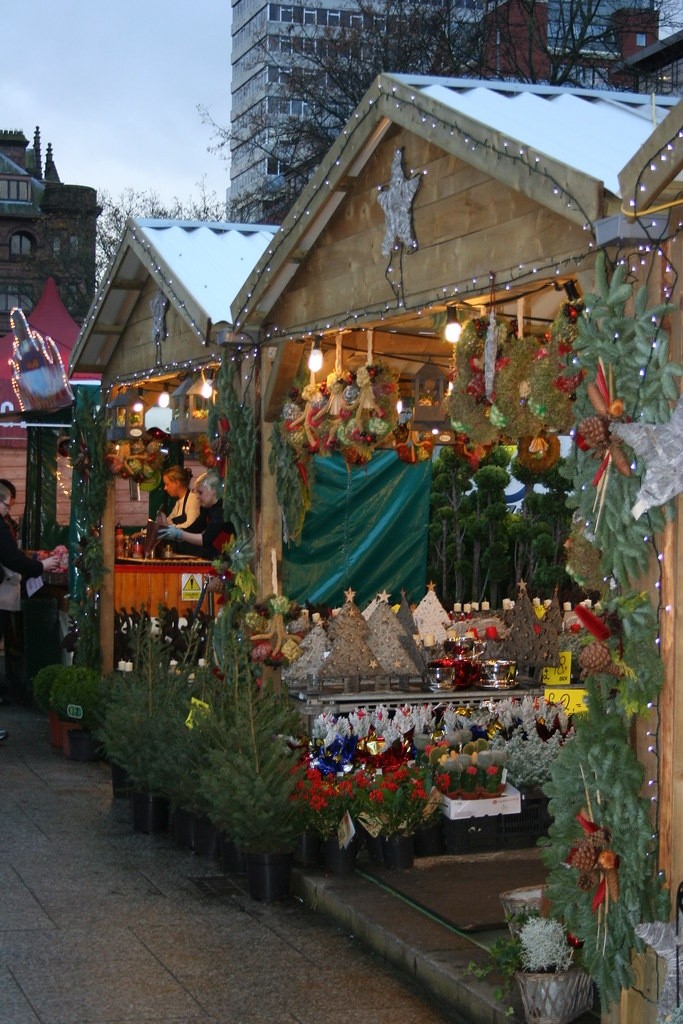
[154,465,238,561]
[0,478,60,744]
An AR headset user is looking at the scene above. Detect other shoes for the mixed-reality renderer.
[0,729,8,740]
[0,698,8,705]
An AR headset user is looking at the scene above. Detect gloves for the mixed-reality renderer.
[157,524,182,544]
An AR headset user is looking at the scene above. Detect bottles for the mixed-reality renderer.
[115,529,173,559]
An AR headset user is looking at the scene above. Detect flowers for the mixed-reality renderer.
[289,692,573,838]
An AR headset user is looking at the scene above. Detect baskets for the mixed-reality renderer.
[499,884,549,941]
[516,969,592,1024]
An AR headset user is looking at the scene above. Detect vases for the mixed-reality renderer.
[381,831,414,870]
[322,830,358,874]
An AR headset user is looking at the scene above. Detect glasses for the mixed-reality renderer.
[0,499,12,511]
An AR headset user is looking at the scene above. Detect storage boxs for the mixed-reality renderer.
[432,780,521,820]
[501,801,555,849]
[441,813,500,855]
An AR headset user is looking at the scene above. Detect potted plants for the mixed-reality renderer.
[32,593,321,904]
[463,907,594,1023]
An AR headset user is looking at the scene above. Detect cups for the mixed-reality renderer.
[477,661,518,689]
[426,667,456,691]
